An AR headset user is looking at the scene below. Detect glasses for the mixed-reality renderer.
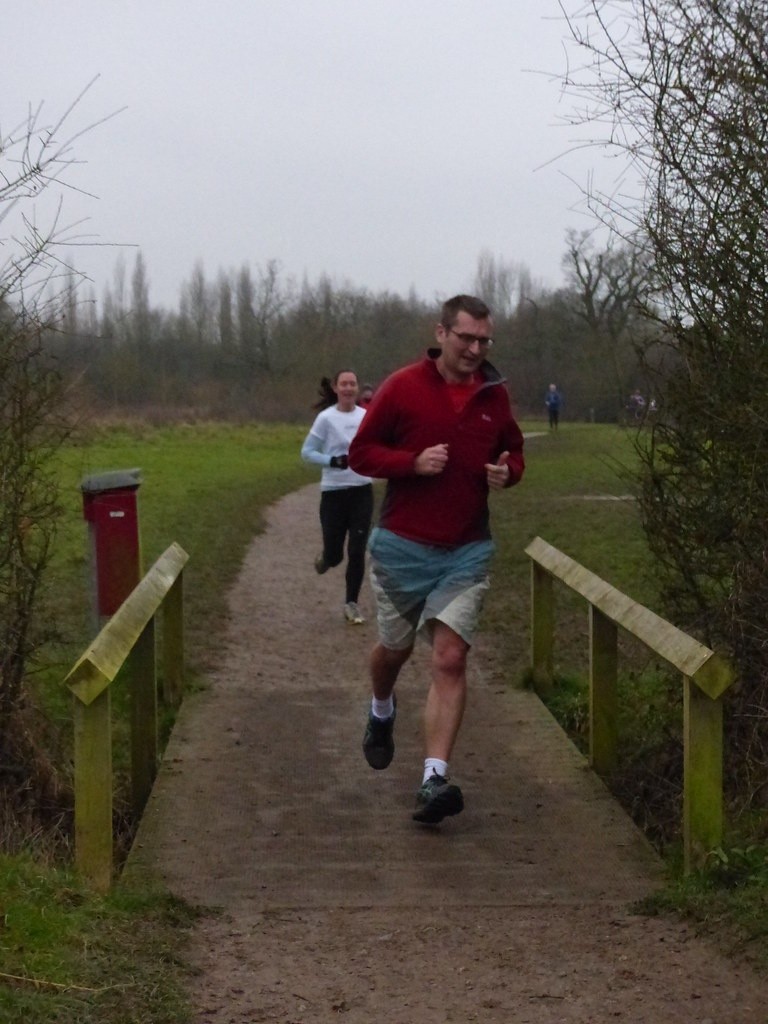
[448,328,494,349]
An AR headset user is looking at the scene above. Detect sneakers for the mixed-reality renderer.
[363,690,402,769]
[413,767,464,825]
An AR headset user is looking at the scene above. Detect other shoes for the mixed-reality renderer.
[346,602,366,623]
[314,552,329,574]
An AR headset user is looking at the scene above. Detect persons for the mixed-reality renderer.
[301,370,375,626]
[624,389,645,427]
[356,383,373,408]
[544,383,561,430]
[347,294,524,825]
[647,397,660,418]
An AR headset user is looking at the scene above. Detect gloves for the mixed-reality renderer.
[330,455,349,470]
